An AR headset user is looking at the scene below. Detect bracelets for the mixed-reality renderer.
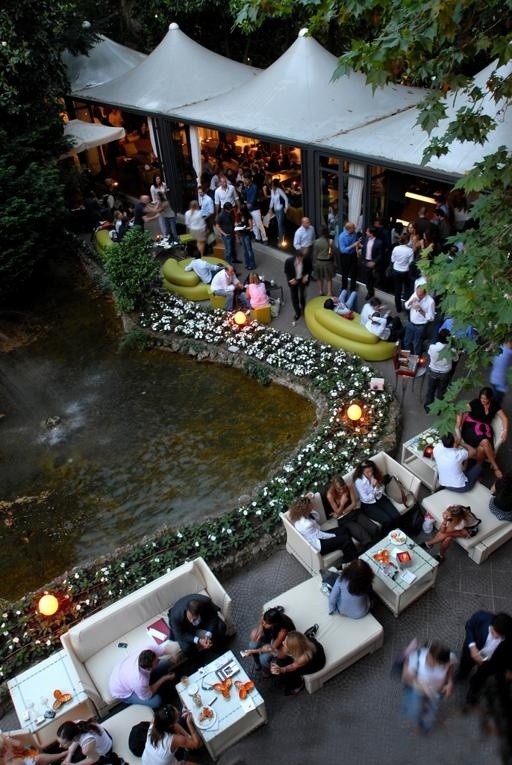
[258,647,262,654]
[284,667,288,672]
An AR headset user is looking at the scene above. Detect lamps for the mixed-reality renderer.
[232,311,247,325]
[35,593,60,617]
[347,403,362,427]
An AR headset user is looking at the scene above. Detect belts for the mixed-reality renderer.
[410,321,428,328]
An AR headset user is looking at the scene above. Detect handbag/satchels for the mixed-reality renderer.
[391,636,429,683]
[304,623,320,638]
[384,477,407,504]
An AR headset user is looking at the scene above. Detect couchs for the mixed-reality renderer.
[160,254,233,301]
[278,453,422,578]
[94,229,117,253]
[58,556,237,718]
[303,295,402,362]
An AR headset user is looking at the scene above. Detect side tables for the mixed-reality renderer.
[401,427,465,494]
[6,649,100,750]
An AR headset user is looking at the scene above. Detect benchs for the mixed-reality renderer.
[420,479,512,566]
[98,702,171,765]
[262,571,385,696]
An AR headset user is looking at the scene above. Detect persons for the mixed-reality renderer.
[168,594,226,669]
[57,718,113,765]
[488,476,512,522]
[285,196,510,415]
[328,558,376,618]
[399,611,512,765]
[419,501,481,561]
[270,632,326,697]
[141,705,201,764]
[109,643,177,710]
[432,432,480,491]
[455,387,509,479]
[0,730,66,764]
[242,608,296,675]
[91,143,301,311]
[288,459,407,564]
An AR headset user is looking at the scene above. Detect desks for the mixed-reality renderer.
[267,171,299,184]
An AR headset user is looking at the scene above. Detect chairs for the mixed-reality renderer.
[458,404,508,469]
[185,240,201,258]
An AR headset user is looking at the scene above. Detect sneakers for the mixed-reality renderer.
[435,554,446,565]
[417,540,434,550]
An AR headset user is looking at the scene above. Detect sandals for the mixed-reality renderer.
[490,464,504,478]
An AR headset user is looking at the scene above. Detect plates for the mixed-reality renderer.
[188,683,198,695]
[388,530,407,546]
[195,706,216,729]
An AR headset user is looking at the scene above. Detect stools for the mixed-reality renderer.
[207,289,226,309]
[249,303,272,327]
[178,234,193,243]
[214,242,226,255]
[282,207,304,226]
